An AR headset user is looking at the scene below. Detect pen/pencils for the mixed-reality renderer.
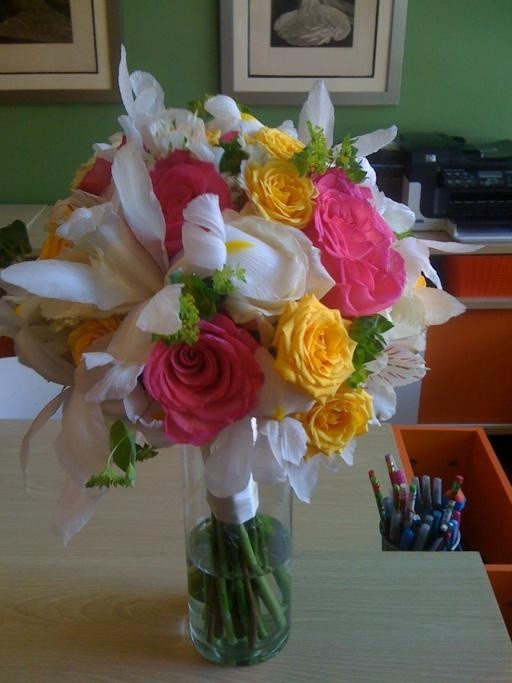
[368,454,466,551]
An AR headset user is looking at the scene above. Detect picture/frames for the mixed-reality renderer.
[219,0,408,106]
[0,0,123,104]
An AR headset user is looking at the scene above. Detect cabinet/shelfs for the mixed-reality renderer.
[414,230,512,436]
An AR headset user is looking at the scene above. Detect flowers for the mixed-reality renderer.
[0,44,485,652]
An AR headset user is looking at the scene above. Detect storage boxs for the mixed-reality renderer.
[440,255,512,299]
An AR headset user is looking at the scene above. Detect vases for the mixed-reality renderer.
[181,417,293,665]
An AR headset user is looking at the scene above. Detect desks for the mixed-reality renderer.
[0,418,512,683]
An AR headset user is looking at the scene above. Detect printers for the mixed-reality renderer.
[401,130,511,246]
